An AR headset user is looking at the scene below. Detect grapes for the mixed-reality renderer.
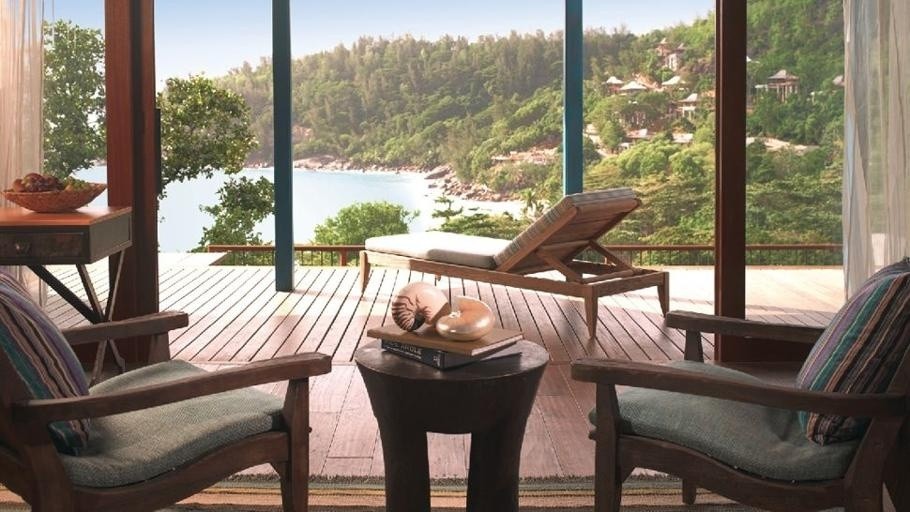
[27,175,90,192]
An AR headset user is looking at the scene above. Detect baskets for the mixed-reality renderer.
[0,182,107,213]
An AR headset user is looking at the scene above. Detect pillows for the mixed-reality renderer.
[0,271,91,455]
[796,257,910,447]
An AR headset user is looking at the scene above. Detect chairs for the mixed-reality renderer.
[359,188,670,339]
[0,311,332,511]
[570,308,910,512]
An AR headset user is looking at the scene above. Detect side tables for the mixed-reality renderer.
[354,338,549,512]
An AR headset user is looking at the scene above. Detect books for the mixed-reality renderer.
[381,339,522,371]
[366,323,524,357]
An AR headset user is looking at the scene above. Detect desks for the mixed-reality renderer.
[0,206,133,387]
